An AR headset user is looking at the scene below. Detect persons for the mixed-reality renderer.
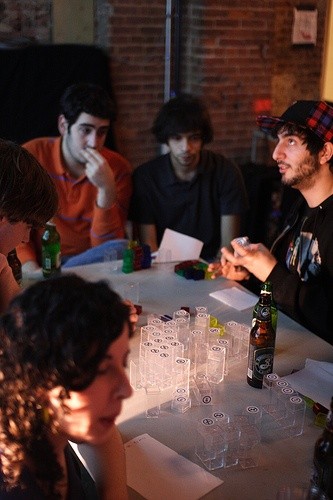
[207,99,333,347]
[15,83,134,286]
[130,93,242,263]
[0,140,59,313]
[0,273,133,500]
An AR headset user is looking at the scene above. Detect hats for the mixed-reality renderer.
[256,100,333,144]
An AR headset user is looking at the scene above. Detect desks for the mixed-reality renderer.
[20,253,332,500]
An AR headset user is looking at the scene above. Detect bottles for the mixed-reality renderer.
[247,292,275,388]
[7,247,23,288]
[42,215,62,278]
[252,281,277,335]
[308,396,333,500]
[122,241,151,272]
